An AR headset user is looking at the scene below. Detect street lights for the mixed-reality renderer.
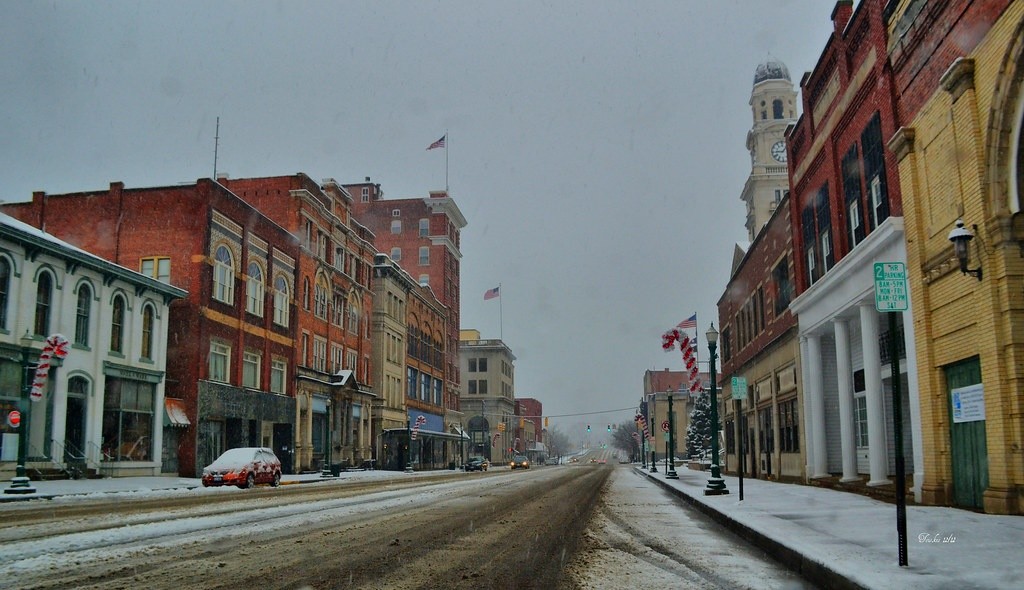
[320,399,334,477]
[404,415,413,473]
[665,385,680,479]
[3,328,38,495]
[705,322,732,495]
[650,410,659,473]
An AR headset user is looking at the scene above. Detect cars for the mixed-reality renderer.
[510,455,531,469]
[202,447,282,489]
[546,457,558,466]
[568,457,606,464]
[466,457,488,472]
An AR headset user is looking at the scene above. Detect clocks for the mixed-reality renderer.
[771,141,788,164]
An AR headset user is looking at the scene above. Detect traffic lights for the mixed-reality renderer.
[608,424,611,431]
[587,424,590,432]
[499,422,502,431]
[502,423,506,432]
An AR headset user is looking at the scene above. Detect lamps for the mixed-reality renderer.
[948,218,983,283]
